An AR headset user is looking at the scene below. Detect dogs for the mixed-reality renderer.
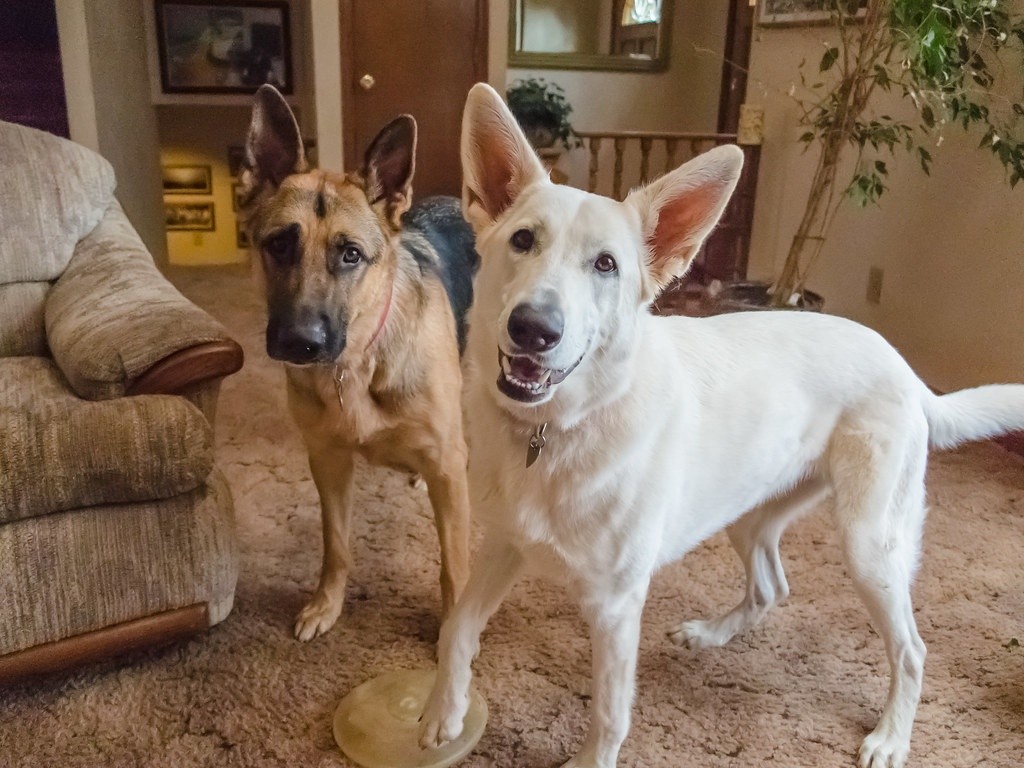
[243,83,482,641]
[419,84,1023,767]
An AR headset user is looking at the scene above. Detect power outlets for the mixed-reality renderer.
[860,264,886,306]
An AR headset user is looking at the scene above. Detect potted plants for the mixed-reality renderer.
[506,78,586,175]
[684,0,1024,319]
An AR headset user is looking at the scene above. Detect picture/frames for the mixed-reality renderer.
[231,183,244,212]
[235,216,251,249]
[164,200,215,231]
[154,0,294,97]
[228,143,245,178]
[160,163,212,194]
[758,0,895,29]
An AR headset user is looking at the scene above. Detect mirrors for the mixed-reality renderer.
[508,0,674,72]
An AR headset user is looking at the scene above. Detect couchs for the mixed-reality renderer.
[0,119,246,690]
